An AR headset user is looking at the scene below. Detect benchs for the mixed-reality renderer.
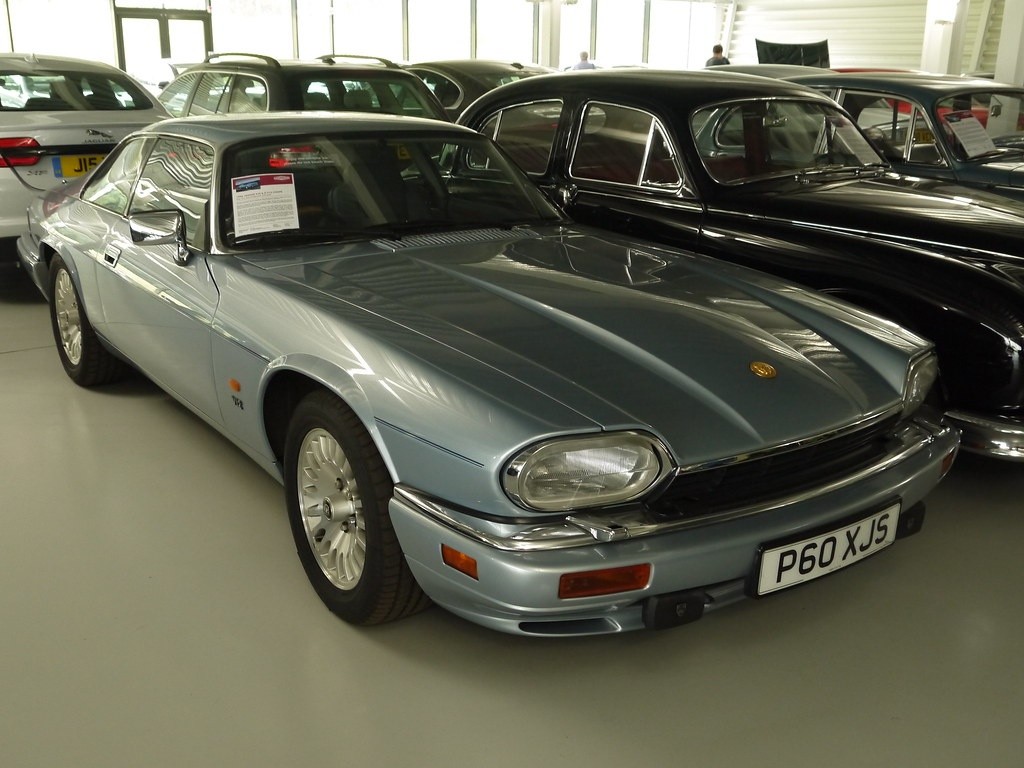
[889,143,939,163]
[581,155,752,184]
[503,141,622,173]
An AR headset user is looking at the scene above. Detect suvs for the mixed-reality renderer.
[397,70,1023,465]
[154,53,454,169]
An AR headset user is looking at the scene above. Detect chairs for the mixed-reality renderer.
[344,90,372,106]
[443,82,460,107]
[290,174,332,206]
[330,177,405,220]
[303,92,329,107]
[86,95,125,108]
[25,94,74,109]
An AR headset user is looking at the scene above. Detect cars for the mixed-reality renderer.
[402,60,608,129]
[18,110,964,642]
[1,52,177,241]
[620,64,1024,204]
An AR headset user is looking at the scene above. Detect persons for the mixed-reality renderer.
[572,49,595,69]
[705,44,729,65]
